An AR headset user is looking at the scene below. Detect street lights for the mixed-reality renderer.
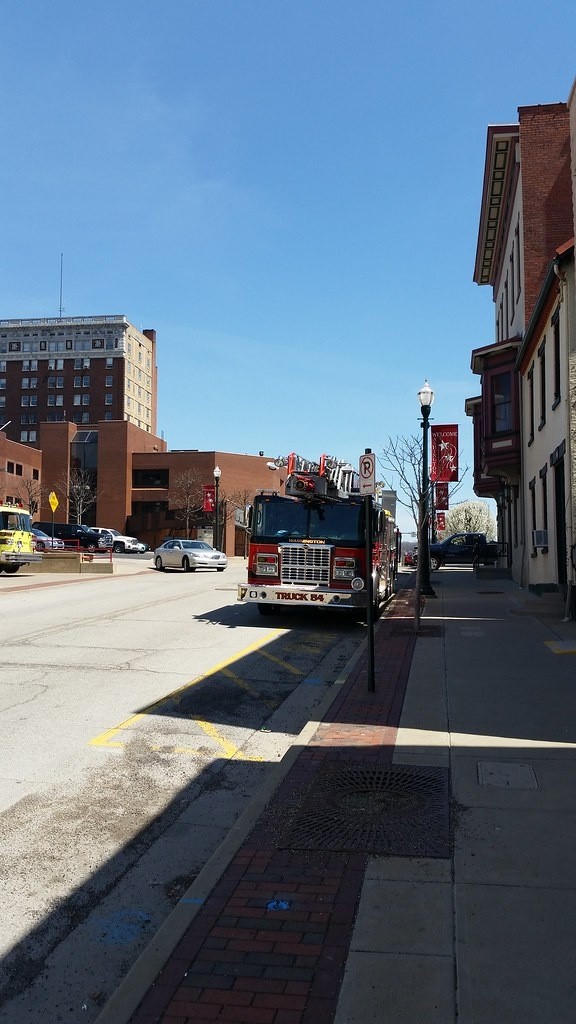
[213,465,222,552]
[416,376,439,598]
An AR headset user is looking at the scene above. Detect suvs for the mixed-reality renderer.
[32,520,108,554]
[86,527,139,554]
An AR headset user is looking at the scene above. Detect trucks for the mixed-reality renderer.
[0,500,44,575]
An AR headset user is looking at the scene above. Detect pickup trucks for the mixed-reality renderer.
[430,532,498,572]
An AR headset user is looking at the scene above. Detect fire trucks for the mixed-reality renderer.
[236,453,402,626]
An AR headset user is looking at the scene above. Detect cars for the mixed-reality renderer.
[153,539,228,573]
[404,550,417,567]
[126,540,152,554]
[31,528,65,552]
